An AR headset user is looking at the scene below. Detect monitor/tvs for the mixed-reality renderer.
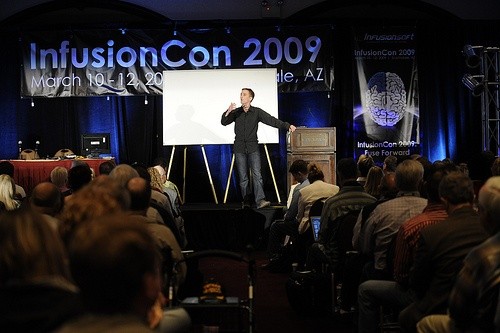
[81,133,110,159]
[310,216,322,241]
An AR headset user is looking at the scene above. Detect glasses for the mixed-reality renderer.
[473,204,483,212]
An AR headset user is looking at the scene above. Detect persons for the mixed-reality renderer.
[135,162,186,249]
[0,210,80,333]
[334,154,398,327]
[270,159,377,310]
[51,166,71,199]
[414,154,500,207]
[110,164,139,184]
[0,174,22,213]
[356,167,451,333]
[67,163,95,194]
[99,160,116,176]
[221,88,297,209]
[30,181,62,232]
[398,174,489,333]
[358,159,428,288]
[56,213,165,333]
[57,176,122,244]
[124,177,187,299]
[415,177,500,333]
[0,161,27,200]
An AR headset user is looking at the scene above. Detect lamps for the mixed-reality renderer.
[461,74,486,97]
[459,45,485,75]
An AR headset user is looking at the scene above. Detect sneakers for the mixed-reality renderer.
[240,202,251,209]
[257,199,270,208]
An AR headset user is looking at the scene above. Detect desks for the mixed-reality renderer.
[0,157,116,196]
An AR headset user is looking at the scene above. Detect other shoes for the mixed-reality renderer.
[262,262,282,271]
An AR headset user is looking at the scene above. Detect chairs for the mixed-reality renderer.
[54,148,75,157]
[19,149,40,159]
[165,249,258,333]
[274,197,451,333]
